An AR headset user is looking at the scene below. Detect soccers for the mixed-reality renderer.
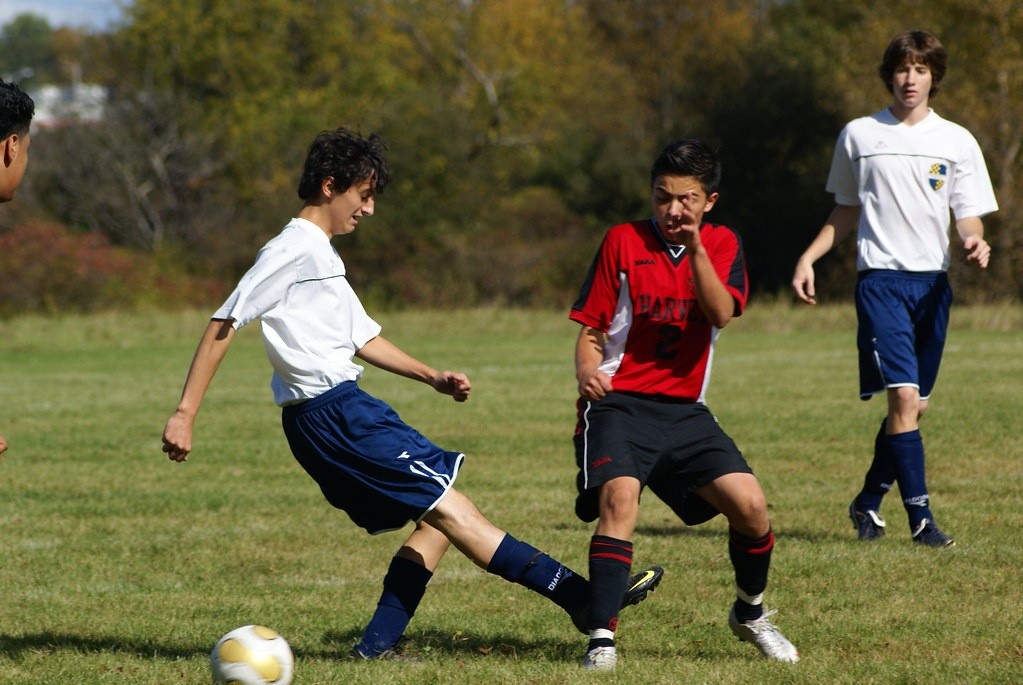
[209,624,294,685]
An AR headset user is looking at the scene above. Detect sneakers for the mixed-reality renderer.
[848,494,885,542]
[583,647,616,672]
[351,635,409,660]
[728,602,800,664]
[573,566,664,635]
[910,517,957,549]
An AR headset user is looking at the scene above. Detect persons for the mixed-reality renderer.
[792,30,999,549]
[0,77,34,452]
[569,140,800,671]
[161,127,664,659]
